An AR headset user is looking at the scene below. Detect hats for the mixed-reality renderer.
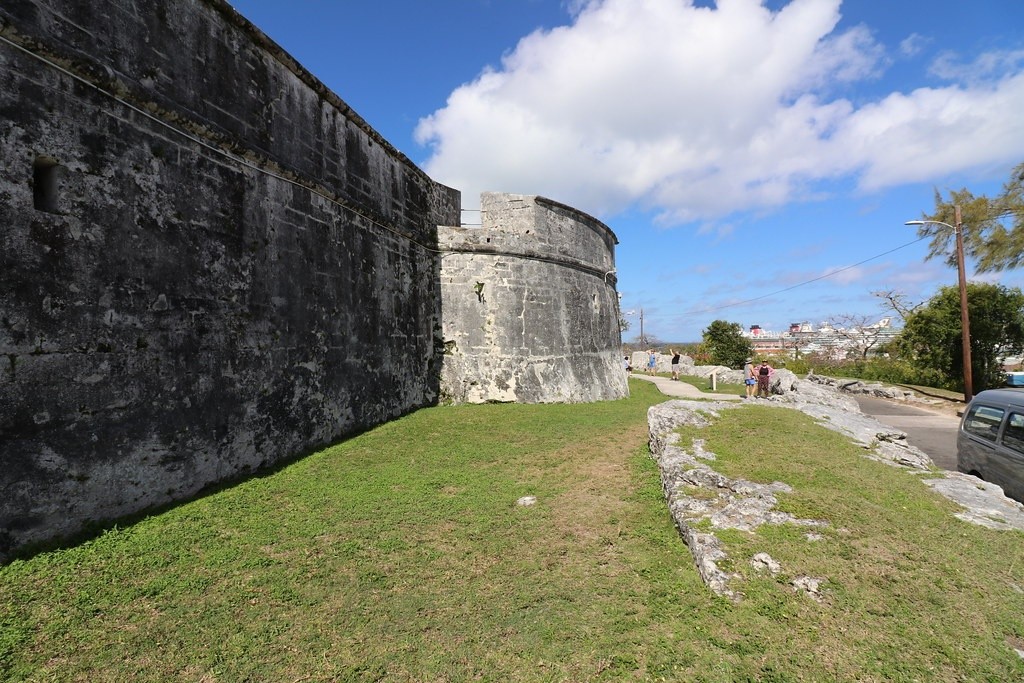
[744,357,753,363]
[762,359,768,363]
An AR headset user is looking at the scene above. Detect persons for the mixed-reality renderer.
[624,356,632,375]
[647,350,656,377]
[744,357,775,398]
[669,348,680,380]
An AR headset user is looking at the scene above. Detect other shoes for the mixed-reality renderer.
[746,395,749,398]
[757,395,760,397]
[675,377,680,380]
[672,376,675,379]
[751,394,754,398]
[766,396,769,398]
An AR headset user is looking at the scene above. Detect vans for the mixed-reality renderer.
[956,388,1024,504]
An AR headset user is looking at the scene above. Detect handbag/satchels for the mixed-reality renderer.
[752,376,759,381]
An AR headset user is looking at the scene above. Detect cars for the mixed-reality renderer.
[1004,371,1024,388]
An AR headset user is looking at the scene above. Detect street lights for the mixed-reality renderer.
[904,204,972,405]
